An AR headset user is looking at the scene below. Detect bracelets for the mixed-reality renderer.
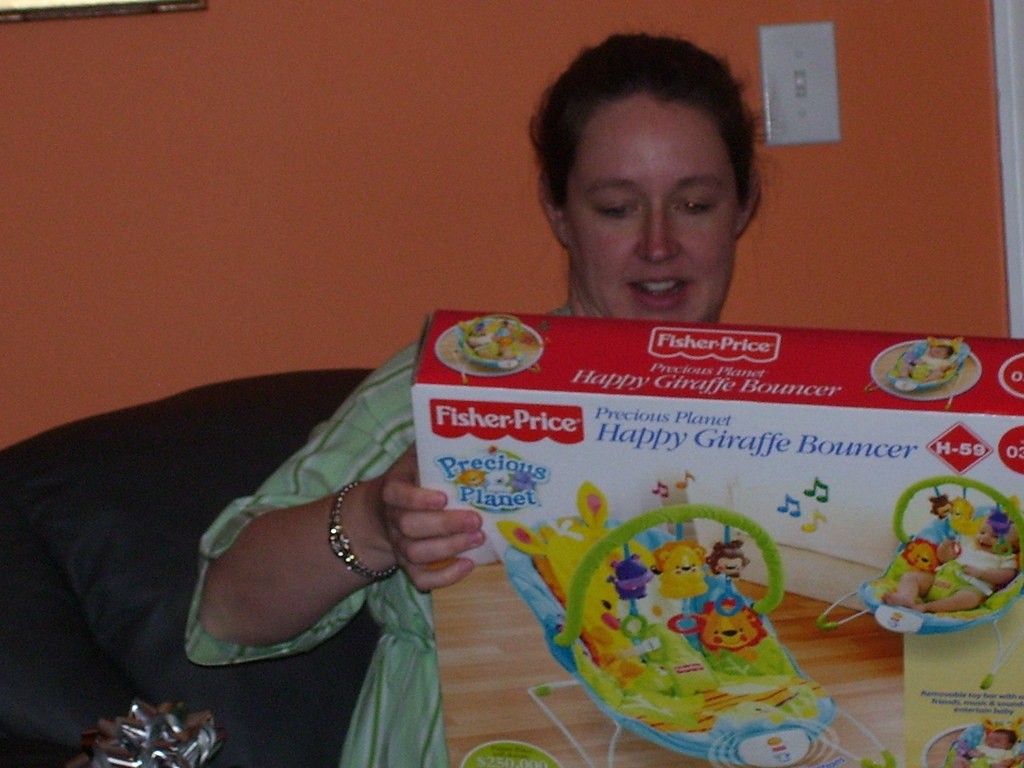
[331,479,399,581]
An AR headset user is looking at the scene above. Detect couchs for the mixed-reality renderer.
[0,368,385,768]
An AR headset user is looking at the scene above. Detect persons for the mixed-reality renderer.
[897,343,954,382]
[952,728,1016,768]
[181,32,780,768]
[880,509,1019,617]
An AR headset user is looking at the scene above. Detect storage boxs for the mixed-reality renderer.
[410,309,1024,768]
